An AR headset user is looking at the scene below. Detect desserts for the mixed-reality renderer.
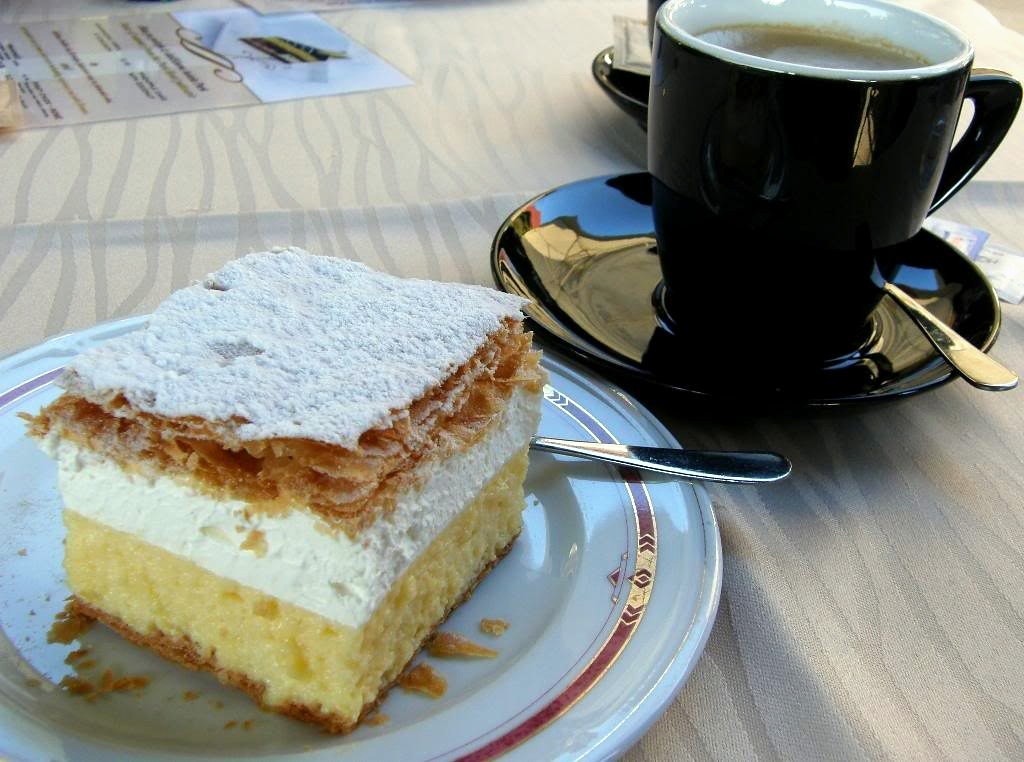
[24,246,549,735]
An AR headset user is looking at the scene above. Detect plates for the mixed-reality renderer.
[489,169,1002,410]
[590,44,648,131]
[0,312,724,762]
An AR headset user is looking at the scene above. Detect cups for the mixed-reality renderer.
[643,0,1024,360]
